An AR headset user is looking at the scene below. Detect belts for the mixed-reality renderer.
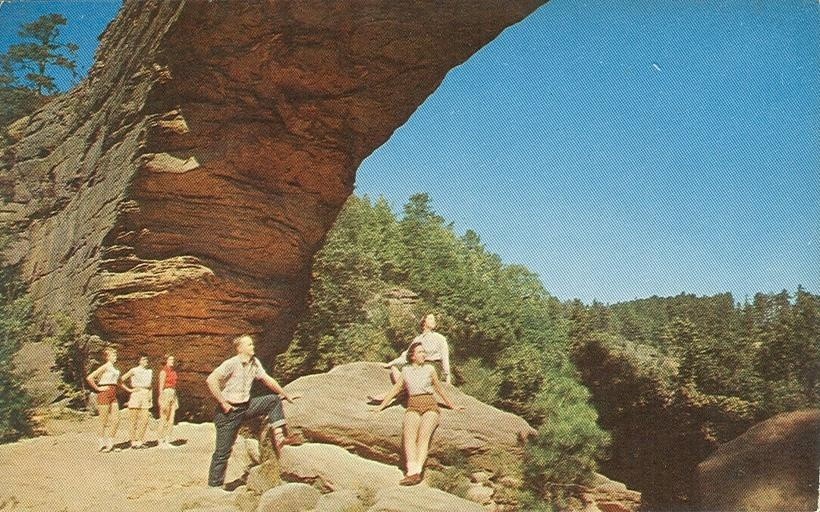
[425,360,441,363]
[98,384,117,387]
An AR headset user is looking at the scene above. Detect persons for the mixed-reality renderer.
[371,341,467,485]
[119,353,154,448]
[367,313,450,403]
[204,334,302,487]
[85,347,121,451]
[157,353,181,449]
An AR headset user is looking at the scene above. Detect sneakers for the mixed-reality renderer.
[399,469,423,486]
[273,431,303,457]
[98,438,178,454]
